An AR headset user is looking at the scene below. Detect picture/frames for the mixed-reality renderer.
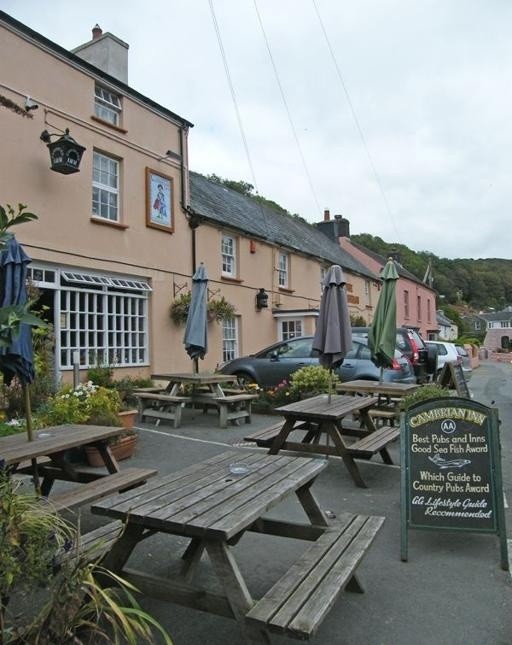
[145,166,174,233]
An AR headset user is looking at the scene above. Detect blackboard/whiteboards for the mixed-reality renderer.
[438,361,451,387]
[401,396,505,532]
[449,362,470,399]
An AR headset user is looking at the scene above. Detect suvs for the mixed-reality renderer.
[352,325,439,385]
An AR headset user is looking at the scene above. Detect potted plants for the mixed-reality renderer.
[82,411,140,468]
[111,381,127,401]
[207,295,236,326]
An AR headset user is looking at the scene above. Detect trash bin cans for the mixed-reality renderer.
[478,348,488,360]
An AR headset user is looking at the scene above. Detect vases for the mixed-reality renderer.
[116,408,138,427]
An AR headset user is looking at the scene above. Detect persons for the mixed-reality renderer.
[156,184,166,220]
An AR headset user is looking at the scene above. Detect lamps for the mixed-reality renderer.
[40,112,87,175]
[256,288,268,313]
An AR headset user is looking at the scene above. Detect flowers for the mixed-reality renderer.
[42,381,121,423]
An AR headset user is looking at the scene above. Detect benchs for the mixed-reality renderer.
[43,519,159,571]
[133,393,191,428]
[132,388,172,413]
[195,386,248,416]
[34,467,158,516]
[211,393,260,428]
[376,396,402,408]
[245,512,386,641]
[351,408,397,428]
[243,419,306,445]
[344,425,400,460]
[11,455,53,472]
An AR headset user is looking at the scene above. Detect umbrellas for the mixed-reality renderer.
[1,232,41,503]
[369,256,399,429]
[311,264,353,458]
[184,261,208,410]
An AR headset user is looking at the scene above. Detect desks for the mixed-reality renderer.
[335,379,423,429]
[151,372,241,428]
[267,393,394,489]
[0,423,129,500]
[79,448,365,645]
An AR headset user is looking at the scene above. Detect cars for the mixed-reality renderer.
[217,334,417,391]
[424,339,472,384]
